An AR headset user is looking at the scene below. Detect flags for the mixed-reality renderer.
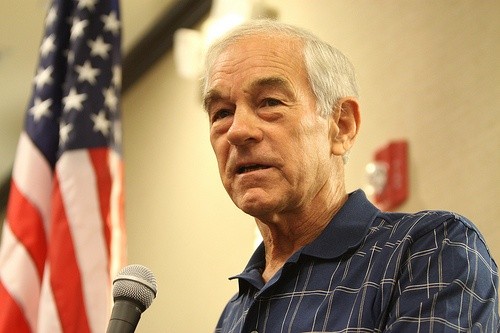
[0,0,124,332]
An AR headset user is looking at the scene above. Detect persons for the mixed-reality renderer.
[198,21,496,333]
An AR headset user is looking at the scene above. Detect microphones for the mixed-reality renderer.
[106,264,157,333]
